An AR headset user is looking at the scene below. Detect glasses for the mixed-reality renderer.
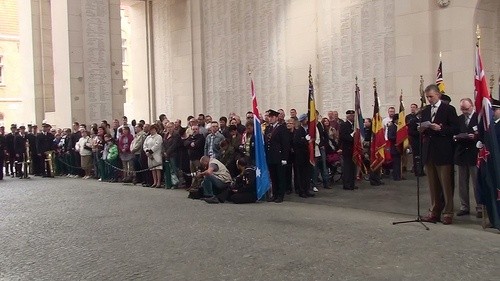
[247,117,253,119]
[460,105,472,111]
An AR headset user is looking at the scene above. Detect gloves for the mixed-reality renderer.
[146,149,153,154]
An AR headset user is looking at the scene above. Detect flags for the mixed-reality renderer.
[352,91,365,169]
[308,90,316,167]
[474,43,492,168]
[435,61,445,94]
[251,80,272,201]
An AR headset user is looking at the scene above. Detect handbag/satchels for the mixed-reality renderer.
[83,138,92,151]
[148,155,159,168]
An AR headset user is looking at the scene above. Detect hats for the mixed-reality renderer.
[268,110,280,117]
[346,110,355,114]
[19,126,25,129]
[491,98,500,110]
[10,124,16,127]
[299,114,308,122]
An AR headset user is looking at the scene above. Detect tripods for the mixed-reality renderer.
[393,98,437,230]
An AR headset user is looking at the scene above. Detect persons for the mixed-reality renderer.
[370,88,386,171]
[417,84,500,232]
[0,104,426,204]
[395,94,409,154]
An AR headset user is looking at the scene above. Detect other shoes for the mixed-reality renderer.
[274,194,283,202]
[421,212,441,222]
[370,180,384,186]
[477,211,482,218]
[267,194,276,202]
[456,210,469,216]
[313,187,318,192]
[295,189,309,198]
[443,214,454,224]
[156,184,160,188]
[325,185,333,189]
[151,184,156,187]
[343,185,359,190]
[54,172,150,187]
